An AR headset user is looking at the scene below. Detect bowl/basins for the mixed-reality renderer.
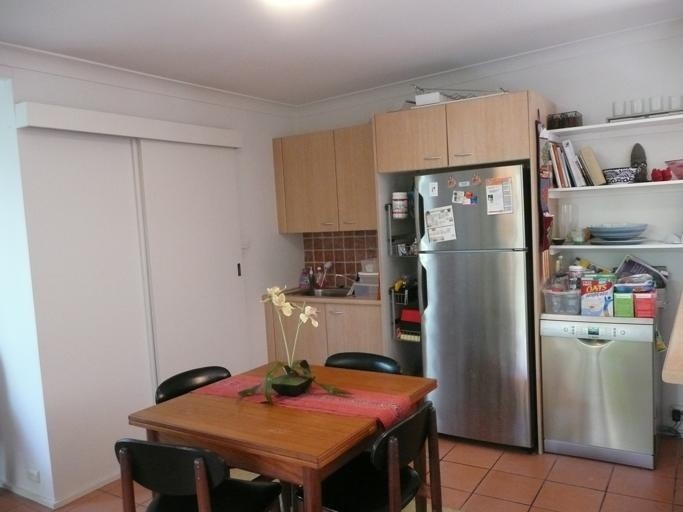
[550,238,566,245]
[588,223,647,240]
[603,167,637,185]
[664,159,683,180]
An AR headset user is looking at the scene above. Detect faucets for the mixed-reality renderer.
[334,274,356,286]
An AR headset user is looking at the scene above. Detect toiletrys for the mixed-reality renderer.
[299,266,324,290]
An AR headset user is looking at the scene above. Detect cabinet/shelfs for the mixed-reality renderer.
[545,113,683,255]
[265,302,383,366]
[372,88,531,174]
[271,123,378,235]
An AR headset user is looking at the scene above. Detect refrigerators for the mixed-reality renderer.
[415,165,532,452]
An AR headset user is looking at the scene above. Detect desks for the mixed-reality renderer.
[128,361,443,511]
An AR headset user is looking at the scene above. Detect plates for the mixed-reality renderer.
[590,237,647,245]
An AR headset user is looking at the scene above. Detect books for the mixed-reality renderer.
[550,138,606,189]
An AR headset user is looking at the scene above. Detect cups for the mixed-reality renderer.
[613,95,671,118]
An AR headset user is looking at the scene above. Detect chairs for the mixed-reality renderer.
[113,436,286,511]
[324,353,404,378]
[316,400,442,509]
[154,364,232,404]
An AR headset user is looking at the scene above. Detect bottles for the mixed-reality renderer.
[314,267,325,288]
[299,272,311,290]
[392,192,408,220]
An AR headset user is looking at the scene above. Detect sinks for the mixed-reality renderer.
[286,289,353,296]
